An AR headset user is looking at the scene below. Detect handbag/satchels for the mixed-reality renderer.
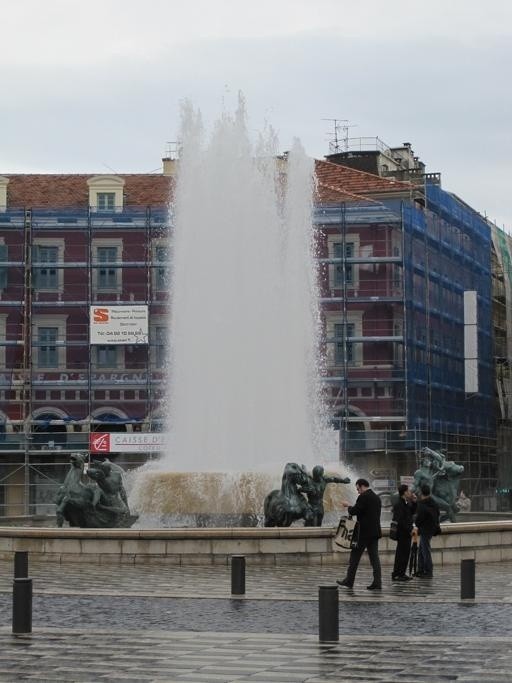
[389,519,400,541]
[332,508,360,549]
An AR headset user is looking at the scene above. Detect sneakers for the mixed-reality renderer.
[391,571,434,581]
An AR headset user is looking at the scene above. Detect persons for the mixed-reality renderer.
[456,490,471,512]
[409,458,457,522]
[298,466,350,527]
[336,479,382,589]
[392,485,441,581]
[86,462,130,514]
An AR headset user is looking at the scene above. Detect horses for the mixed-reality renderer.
[51,451,127,514]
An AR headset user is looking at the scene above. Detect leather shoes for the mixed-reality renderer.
[366,582,384,589]
[336,578,355,587]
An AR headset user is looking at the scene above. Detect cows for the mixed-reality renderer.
[432,459,465,523]
